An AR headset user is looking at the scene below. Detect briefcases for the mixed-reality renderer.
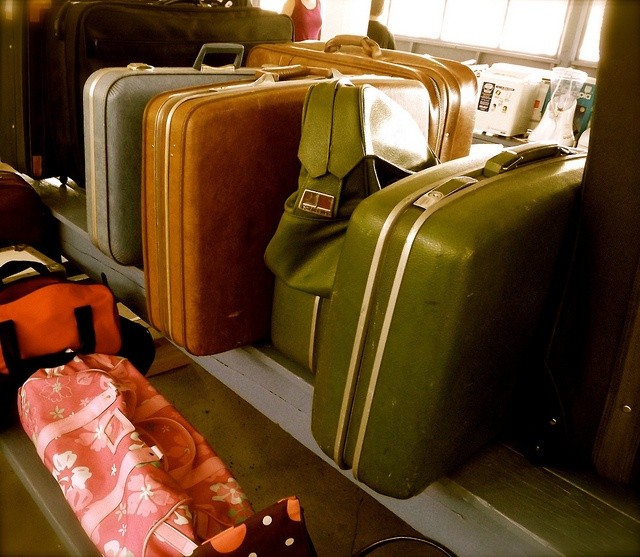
[41,1,296,186]
[249,34,476,165]
[80,41,263,266]
[308,141,587,499]
[140,65,437,358]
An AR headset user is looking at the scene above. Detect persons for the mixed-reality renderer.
[280,0,323,41]
[366,0,396,49]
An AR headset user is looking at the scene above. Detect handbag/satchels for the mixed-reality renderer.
[1,259,122,427]
[271,277,333,374]
[16,351,253,557]
[260,80,438,299]
[187,494,316,557]
[0,167,62,256]
